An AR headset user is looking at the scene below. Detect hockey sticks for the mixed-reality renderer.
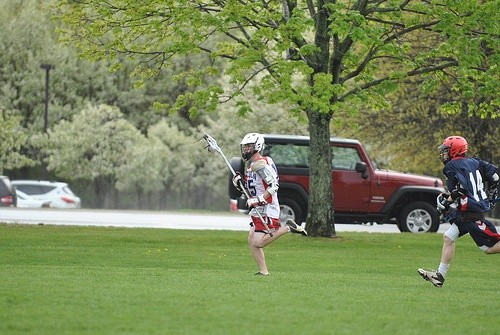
[203,132,274,238]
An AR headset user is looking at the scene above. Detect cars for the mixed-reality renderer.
[0,175,18,207]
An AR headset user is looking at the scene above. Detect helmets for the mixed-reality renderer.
[437,135,469,163]
[239,132,265,160]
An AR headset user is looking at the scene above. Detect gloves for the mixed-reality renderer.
[487,188,498,209]
[244,194,269,211]
[231,171,245,192]
[435,192,451,214]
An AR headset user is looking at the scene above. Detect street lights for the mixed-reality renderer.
[40,61,56,133]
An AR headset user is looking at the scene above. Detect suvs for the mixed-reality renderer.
[225,134,450,233]
[10,180,82,210]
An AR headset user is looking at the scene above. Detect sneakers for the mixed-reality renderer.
[416,267,445,289]
[253,270,271,279]
[286,218,308,238]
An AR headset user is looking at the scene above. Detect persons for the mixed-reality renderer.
[232,132,308,277]
[417,136,500,288]
